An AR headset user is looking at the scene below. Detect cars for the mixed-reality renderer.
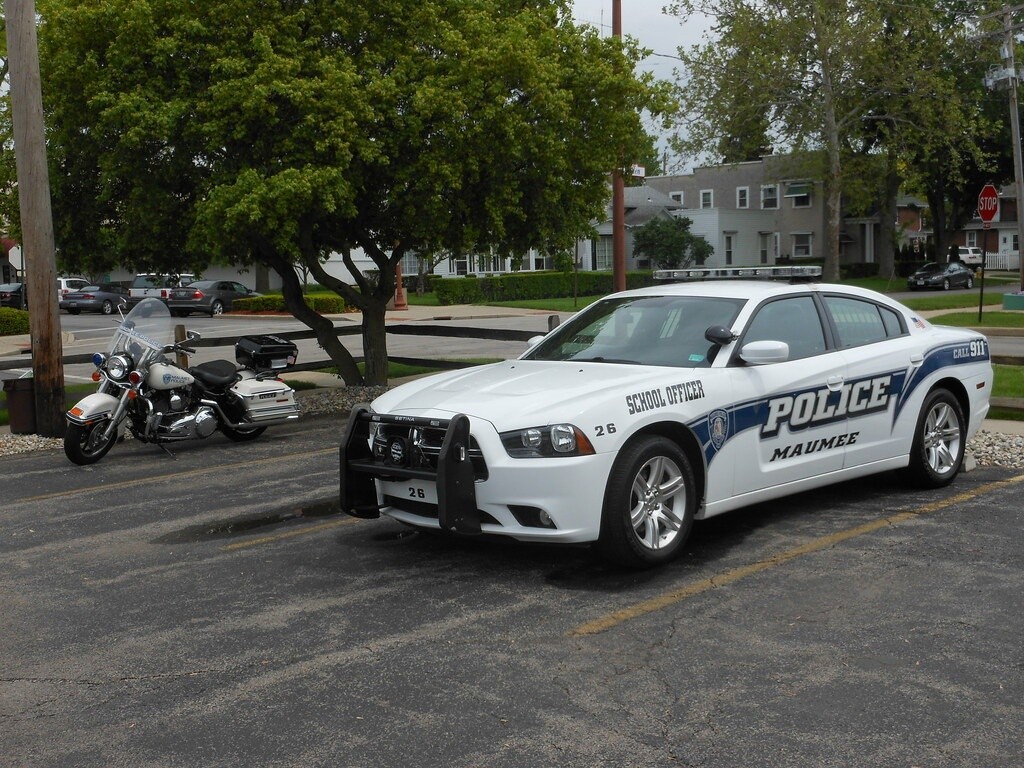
[167,279,264,318]
[60,283,130,315]
[907,260,975,291]
[337,264,995,579]
[0,282,28,311]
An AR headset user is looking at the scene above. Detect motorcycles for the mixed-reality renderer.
[63,297,301,466]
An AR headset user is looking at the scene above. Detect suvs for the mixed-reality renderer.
[57,277,92,304]
[127,272,198,318]
[947,246,987,273]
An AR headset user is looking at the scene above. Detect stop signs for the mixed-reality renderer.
[978,184,999,223]
